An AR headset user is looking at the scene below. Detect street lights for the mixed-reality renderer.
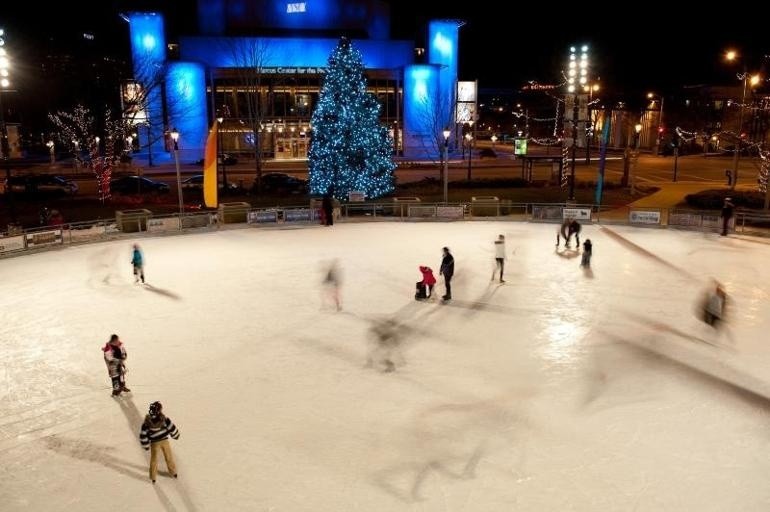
[168,129,184,216]
[564,41,591,202]
[441,125,451,204]
[216,112,230,200]
[252,171,309,194]
[647,91,666,154]
[0,26,16,185]
[723,44,762,189]
[583,83,600,164]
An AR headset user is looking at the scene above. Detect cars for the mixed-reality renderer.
[472,145,498,160]
[197,154,235,166]
[178,174,238,196]
[110,174,170,196]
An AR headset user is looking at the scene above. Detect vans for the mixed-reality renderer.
[3,174,80,198]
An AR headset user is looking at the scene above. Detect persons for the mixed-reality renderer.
[440,247,454,300]
[491,234,508,283]
[312,253,401,377]
[130,244,145,283]
[694,278,731,349]
[554,217,591,267]
[101,334,131,397]
[720,197,736,235]
[48,209,68,230]
[140,401,180,482]
[420,266,436,298]
[320,194,341,225]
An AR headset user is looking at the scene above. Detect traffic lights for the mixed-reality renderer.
[658,125,664,139]
